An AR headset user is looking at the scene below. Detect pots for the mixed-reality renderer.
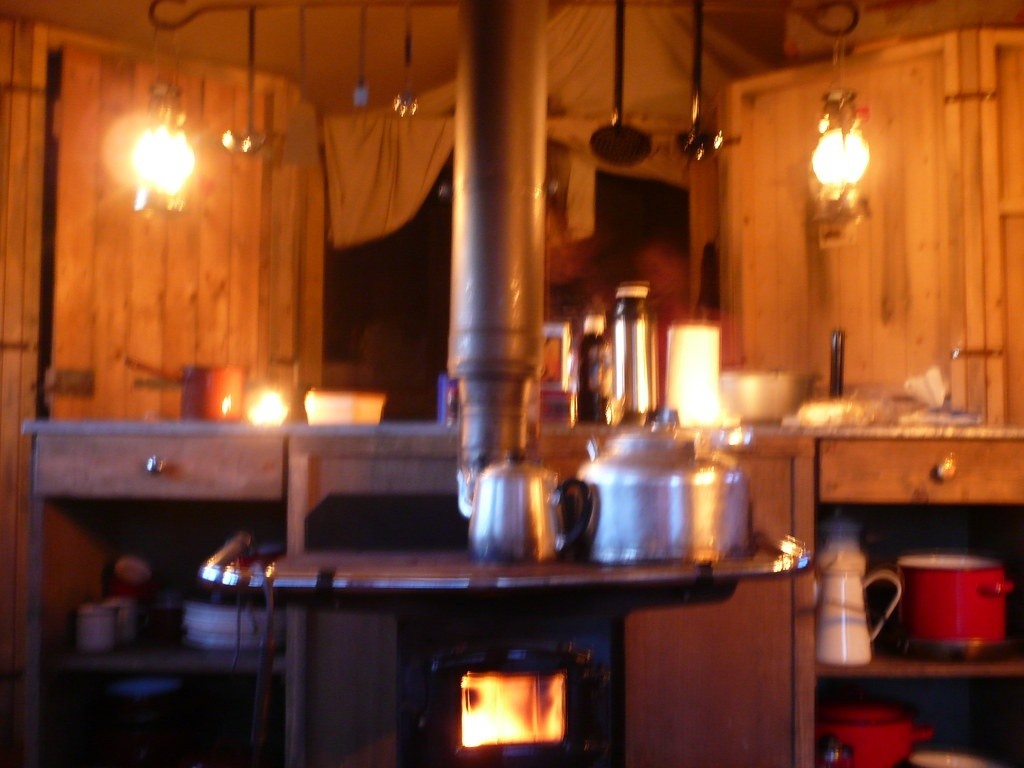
[819,711,931,768]
[126,357,240,422]
[895,555,1013,657]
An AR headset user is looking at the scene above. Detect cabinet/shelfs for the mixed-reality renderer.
[19,423,302,768]
[820,414,1022,767]
[283,418,821,768]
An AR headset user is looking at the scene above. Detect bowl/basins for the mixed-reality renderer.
[306,390,386,427]
[720,370,822,422]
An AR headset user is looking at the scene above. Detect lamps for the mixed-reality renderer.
[810,29,871,224]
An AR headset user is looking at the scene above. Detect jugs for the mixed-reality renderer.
[813,521,902,667]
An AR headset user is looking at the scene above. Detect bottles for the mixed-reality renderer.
[611,282,657,426]
[577,316,612,422]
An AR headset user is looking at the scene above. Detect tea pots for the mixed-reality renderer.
[456,449,590,571]
[573,413,751,568]
